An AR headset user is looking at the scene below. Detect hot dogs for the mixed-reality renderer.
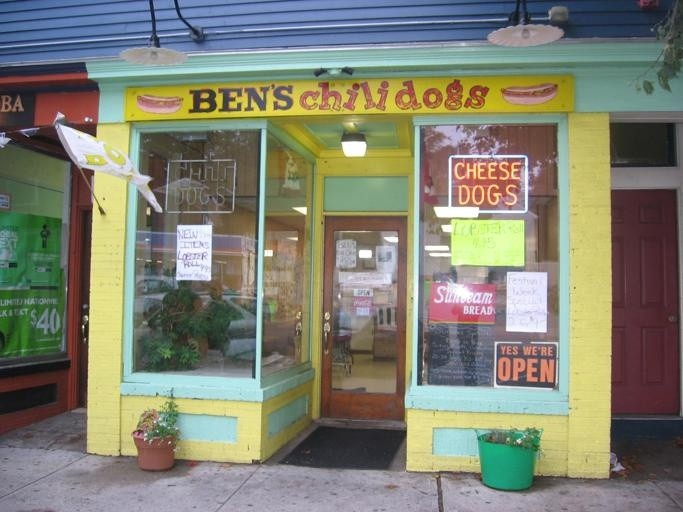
[501,83,559,105]
[136,94,184,113]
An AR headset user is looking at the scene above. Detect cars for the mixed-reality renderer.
[135,275,270,362]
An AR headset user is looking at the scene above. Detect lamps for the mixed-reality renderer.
[487,0,568,47]
[340,130,367,158]
[119,0,203,66]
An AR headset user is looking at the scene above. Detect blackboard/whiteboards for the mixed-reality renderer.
[428,321,497,388]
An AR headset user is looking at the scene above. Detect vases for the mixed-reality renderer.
[131,429,176,471]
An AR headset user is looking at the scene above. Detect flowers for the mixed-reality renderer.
[136,400,183,453]
[505,426,543,452]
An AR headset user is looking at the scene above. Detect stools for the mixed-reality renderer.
[332,327,353,374]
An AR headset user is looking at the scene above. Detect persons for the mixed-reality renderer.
[162,281,202,314]
[449,264,495,385]
[201,278,229,352]
